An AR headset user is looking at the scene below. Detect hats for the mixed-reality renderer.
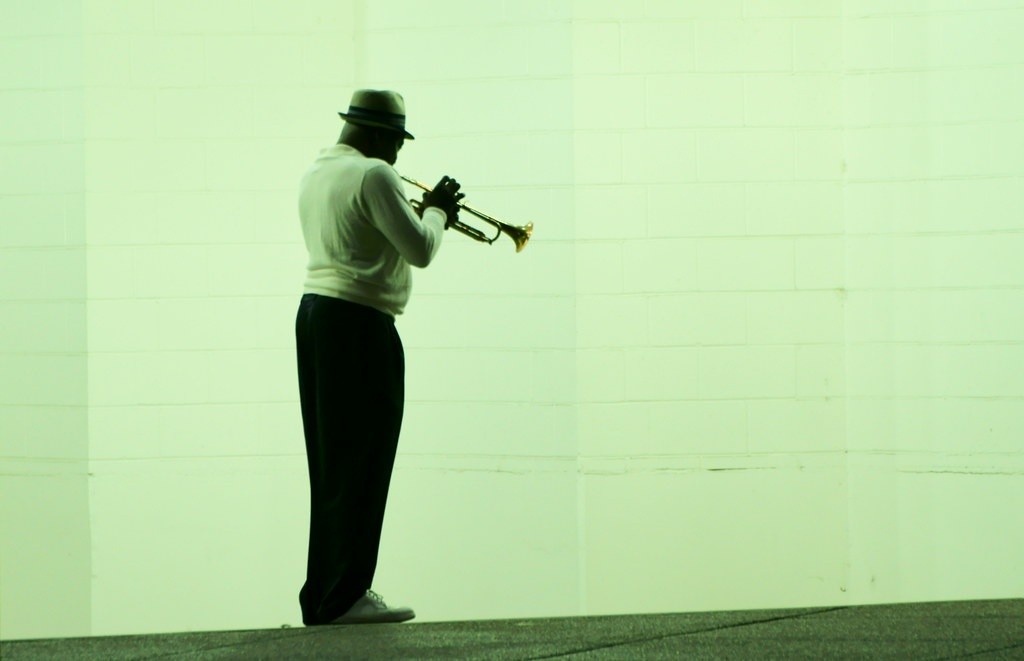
[338,89,414,141]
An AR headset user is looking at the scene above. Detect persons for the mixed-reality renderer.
[295,89,466,628]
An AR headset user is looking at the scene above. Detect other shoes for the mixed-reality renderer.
[334,589,416,623]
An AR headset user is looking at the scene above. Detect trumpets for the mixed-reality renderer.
[391,176,535,256]
